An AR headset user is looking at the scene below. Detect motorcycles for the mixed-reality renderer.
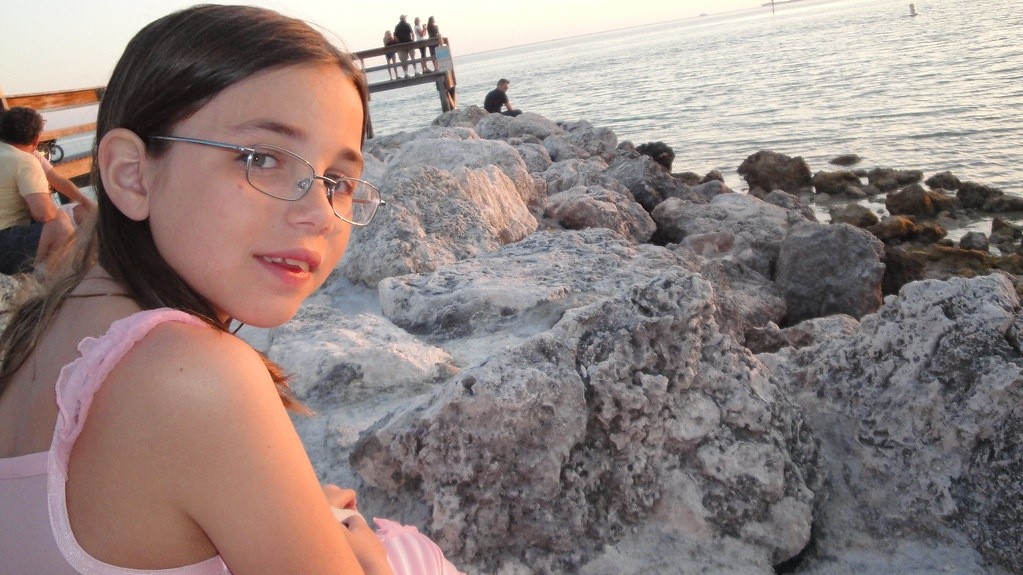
[33,139,64,164]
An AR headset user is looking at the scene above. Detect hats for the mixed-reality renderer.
[399,14,407,19]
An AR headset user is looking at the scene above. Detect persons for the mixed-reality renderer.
[0,4,461,575]
[483,79,522,117]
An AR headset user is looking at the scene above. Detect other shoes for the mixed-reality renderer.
[396,76,400,79]
[422,69,432,74]
[415,72,421,75]
[404,74,410,78]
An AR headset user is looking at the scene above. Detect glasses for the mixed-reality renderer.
[502,85,508,90]
[140,135,386,227]
